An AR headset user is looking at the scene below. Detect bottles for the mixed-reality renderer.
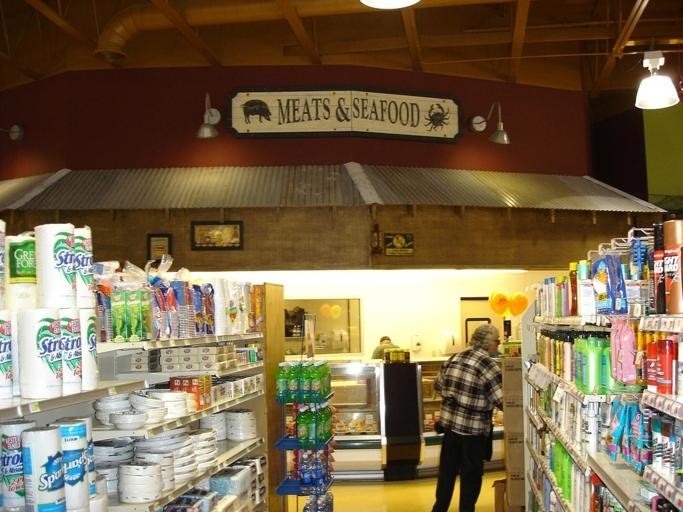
[273,358,337,511]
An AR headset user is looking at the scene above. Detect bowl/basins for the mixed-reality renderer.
[88,385,189,431]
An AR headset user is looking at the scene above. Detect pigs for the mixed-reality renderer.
[240,100,271,124]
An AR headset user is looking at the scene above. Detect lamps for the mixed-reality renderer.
[371,224,383,253]
[469,102,510,145]
[634,50,681,111]
[194,92,221,139]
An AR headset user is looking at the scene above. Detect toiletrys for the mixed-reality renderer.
[525,220,682,512]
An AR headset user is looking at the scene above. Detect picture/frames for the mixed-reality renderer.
[191,222,243,251]
[147,233,172,263]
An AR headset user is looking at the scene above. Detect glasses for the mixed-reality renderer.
[491,337,501,346]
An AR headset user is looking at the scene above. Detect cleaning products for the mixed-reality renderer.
[276,358,335,512]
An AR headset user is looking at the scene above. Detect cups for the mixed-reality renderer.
[94,277,250,344]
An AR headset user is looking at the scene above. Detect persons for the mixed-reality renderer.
[430,323,504,511]
[369,334,401,360]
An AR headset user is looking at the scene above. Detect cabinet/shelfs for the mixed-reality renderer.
[525,316,682,512]
[0,331,266,511]
[331,362,443,481]
[275,391,336,512]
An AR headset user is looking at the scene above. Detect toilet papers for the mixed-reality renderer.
[0,216,98,512]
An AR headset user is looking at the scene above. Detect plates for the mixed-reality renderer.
[94,427,219,507]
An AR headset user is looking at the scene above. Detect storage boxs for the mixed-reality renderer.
[492,357,525,512]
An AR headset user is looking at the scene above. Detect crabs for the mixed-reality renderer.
[424,104,449,131]
